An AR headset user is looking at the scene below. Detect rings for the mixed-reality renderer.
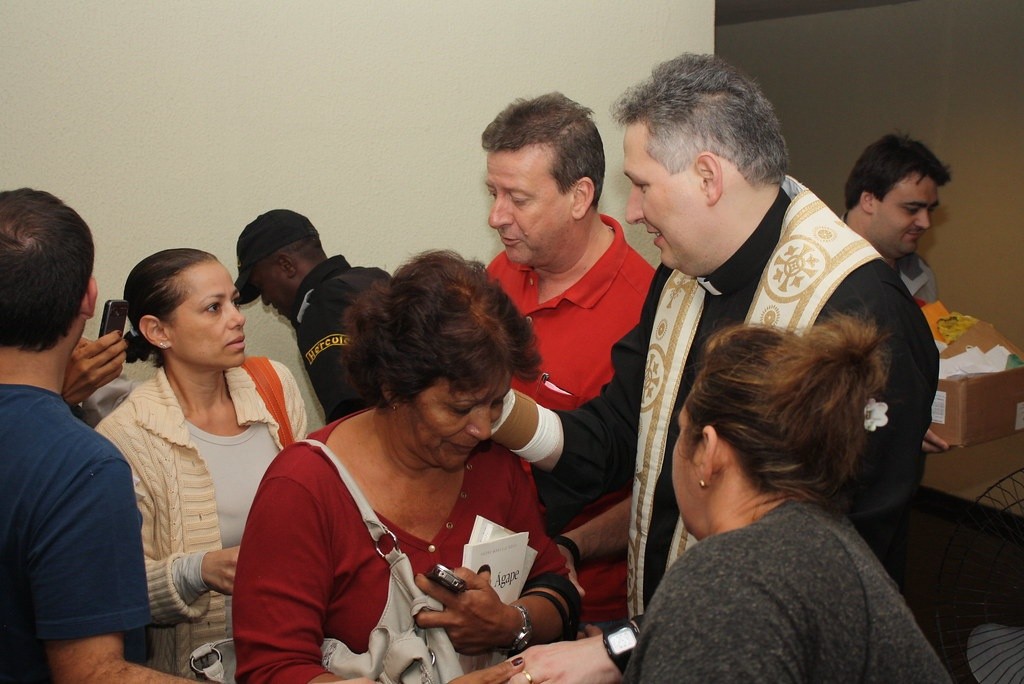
[523,671,533,684]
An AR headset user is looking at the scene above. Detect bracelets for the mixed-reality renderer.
[556,536,581,570]
[521,591,571,632]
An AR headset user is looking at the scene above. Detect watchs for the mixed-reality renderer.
[602,621,640,675]
[500,604,534,653]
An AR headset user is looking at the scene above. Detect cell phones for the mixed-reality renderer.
[99,299,129,339]
[427,564,466,594]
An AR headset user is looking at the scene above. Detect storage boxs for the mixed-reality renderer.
[928,367,1024,447]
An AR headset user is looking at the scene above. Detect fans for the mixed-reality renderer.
[938,467,1024,684]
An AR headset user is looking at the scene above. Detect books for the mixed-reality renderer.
[457,513,538,674]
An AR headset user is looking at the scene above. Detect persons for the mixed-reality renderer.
[0,187,212,684]
[459,564,492,673]
[92,249,309,681]
[490,53,941,684]
[234,210,398,428]
[478,92,657,640]
[232,251,582,684]
[575,318,955,684]
[831,133,955,587]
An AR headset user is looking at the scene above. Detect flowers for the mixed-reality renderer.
[864,398,891,432]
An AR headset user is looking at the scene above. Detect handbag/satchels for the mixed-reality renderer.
[191,438,462,684]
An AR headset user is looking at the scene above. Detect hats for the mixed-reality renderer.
[233,209,320,305]
